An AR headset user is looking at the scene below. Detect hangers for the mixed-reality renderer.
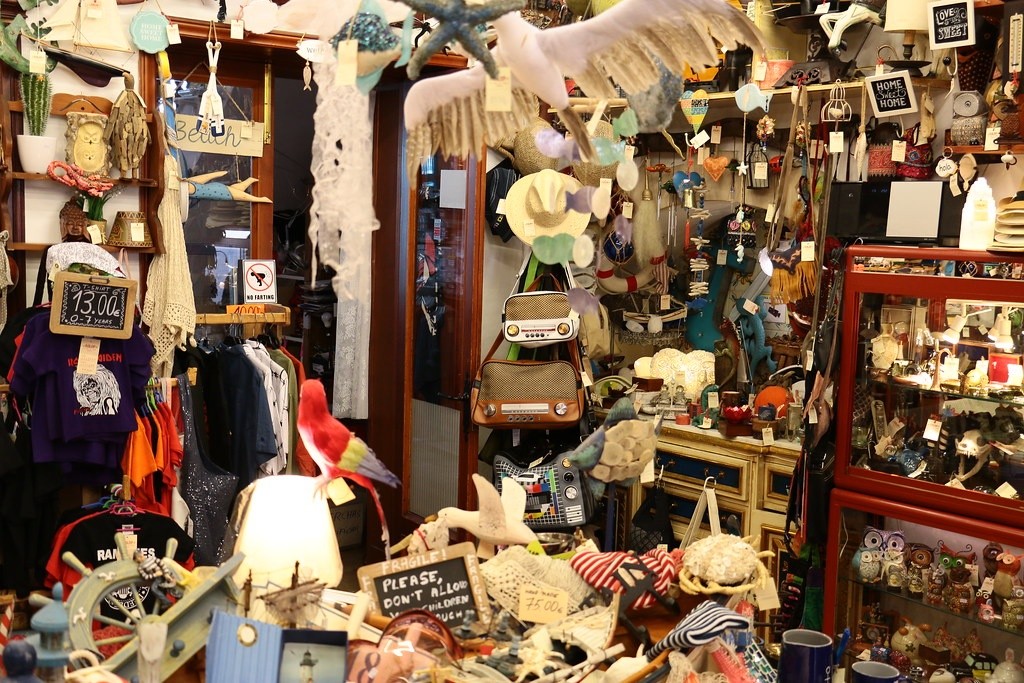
[196,308,285,351]
[82,483,146,532]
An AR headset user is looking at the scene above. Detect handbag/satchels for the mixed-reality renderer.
[574,277,610,359]
[501,290,581,349]
[468,359,598,529]
[768,237,820,306]
[784,259,851,630]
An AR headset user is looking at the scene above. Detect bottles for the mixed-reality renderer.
[959,176,997,251]
[412,181,460,316]
[648,315,663,334]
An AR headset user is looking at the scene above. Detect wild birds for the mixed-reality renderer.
[425,474,540,545]
[297,379,403,571]
[404,0,770,192]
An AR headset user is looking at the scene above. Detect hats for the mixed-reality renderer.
[485,167,522,244]
[505,168,591,246]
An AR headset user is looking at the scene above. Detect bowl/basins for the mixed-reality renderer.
[535,532,574,555]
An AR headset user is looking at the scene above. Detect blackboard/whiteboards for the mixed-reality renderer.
[866,70,918,118]
[358,541,491,638]
[49,271,138,338]
[926,0,976,51]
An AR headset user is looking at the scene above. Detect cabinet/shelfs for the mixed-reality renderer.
[589,247,1024,683]
[1,91,168,257]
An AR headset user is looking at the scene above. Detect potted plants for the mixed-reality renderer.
[17,71,58,174]
[83,183,129,242]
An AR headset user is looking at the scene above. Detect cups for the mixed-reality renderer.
[851,660,901,683]
[776,629,833,683]
[720,391,740,412]
